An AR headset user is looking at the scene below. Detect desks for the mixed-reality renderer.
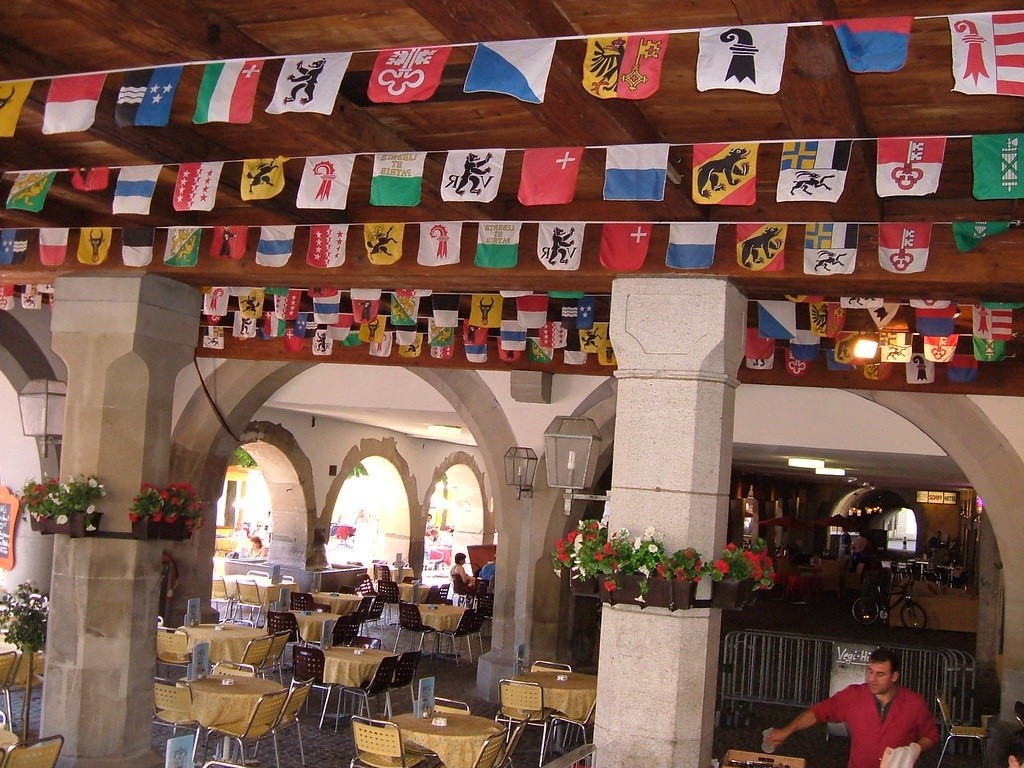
[777,571,826,603]
[321,646,397,716]
[221,575,299,607]
[367,563,414,583]
[175,624,268,664]
[176,676,283,764]
[397,582,431,603]
[385,713,505,768]
[214,537,239,556]
[414,603,468,658]
[307,592,363,616]
[336,525,356,546]
[916,561,928,578]
[511,671,597,755]
[289,609,343,642]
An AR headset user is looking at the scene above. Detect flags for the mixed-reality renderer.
[0,11,1024,385]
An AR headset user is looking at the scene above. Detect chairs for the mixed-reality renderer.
[1014,701,1024,727]
[937,695,991,768]
[0,650,64,768]
[152,560,597,768]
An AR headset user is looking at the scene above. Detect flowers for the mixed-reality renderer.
[551,518,776,604]
[129,482,206,532]
[19,472,106,531]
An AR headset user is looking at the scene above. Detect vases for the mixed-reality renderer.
[711,579,759,610]
[132,515,192,539]
[570,564,596,597]
[596,569,698,609]
[30,511,103,538]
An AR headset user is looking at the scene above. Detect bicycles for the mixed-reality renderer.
[852,577,928,630]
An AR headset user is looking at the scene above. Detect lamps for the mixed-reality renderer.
[18,380,67,458]
[543,415,611,515]
[503,447,538,501]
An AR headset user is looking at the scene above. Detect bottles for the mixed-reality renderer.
[190,617,198,627]
[197,669,206,682]
[423,705,432,718]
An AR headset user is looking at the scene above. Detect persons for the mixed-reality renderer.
[159,552,179,620]
[478,554,496,586]
[768,648,940,768]
[928,531,942,558]
[781,527,882,595]
[1004,726,1024,768]
[450,553,476,593]
[354,509,367,525]
[425,514,441,542]
[229,520,269,557]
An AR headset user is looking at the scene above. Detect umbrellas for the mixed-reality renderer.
[758,515,817,547]
[813,514,866,531]
